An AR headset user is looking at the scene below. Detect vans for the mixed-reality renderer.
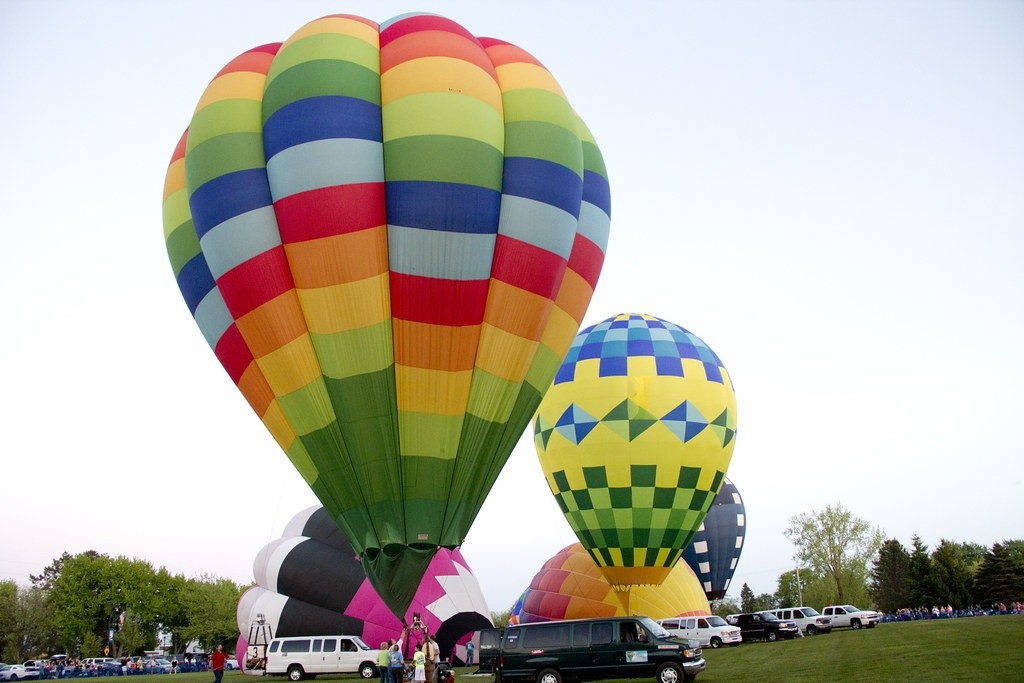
[725,612,798,643]
[265,635,384,681]
[655,615,742,650]
[756,607,833,637]
[475,613,707,683]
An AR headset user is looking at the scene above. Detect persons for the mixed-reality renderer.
[421,635,435,663]
[388,645,406,683]
[39,651,207,680]
[411,645,427,683]
[208,644,236,683]
[377,642,391,683]
[876,601,1024,619]
[429,635,440,664]
[465,638,475,667]
[387,627,406,657]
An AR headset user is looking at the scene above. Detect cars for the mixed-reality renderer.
[0,649,240,683]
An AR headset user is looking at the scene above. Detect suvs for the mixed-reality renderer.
[822,605,880,631]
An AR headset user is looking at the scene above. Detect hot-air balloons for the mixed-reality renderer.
[531,311,739,621]
[509,541,711,637]
[228,504,498,674]
[681,475,747,615]
[158,12,613,683]
